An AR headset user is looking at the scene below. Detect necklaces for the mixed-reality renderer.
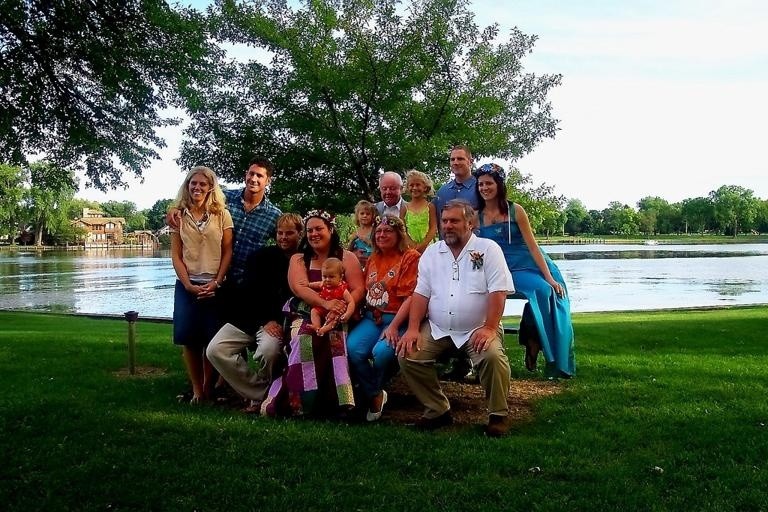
[483,206,500,225]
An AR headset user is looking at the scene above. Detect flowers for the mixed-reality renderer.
[481,163,507,181]
[468,250,485,271]
[375,215,396,227]
[301,209,338,226]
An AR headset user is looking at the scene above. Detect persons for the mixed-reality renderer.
[259,209,367,427]
[398,168,436,254]
[206,211,303,414]
[297,256,355,338]
[426,143,481,385]
[344,199,379,267]
[471,160,577,380]
[346,214,421,423]
[346,170,406,264]
[166,156,284,404]
[393,196,516,437]
[164,166,233,408]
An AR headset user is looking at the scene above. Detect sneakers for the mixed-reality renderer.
[215,387,228,402]
[415,410,452,429]
[488,415,511,434]
[445,361,473,380]
[240,403,260,412]
[191,393,202,405]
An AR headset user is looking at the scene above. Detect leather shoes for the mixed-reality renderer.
[526,346,536,374]
[366,390,388,422]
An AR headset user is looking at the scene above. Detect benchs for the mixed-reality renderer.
[500,291,531,335]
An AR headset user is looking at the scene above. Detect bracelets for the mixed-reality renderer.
[214,278,223,289]
